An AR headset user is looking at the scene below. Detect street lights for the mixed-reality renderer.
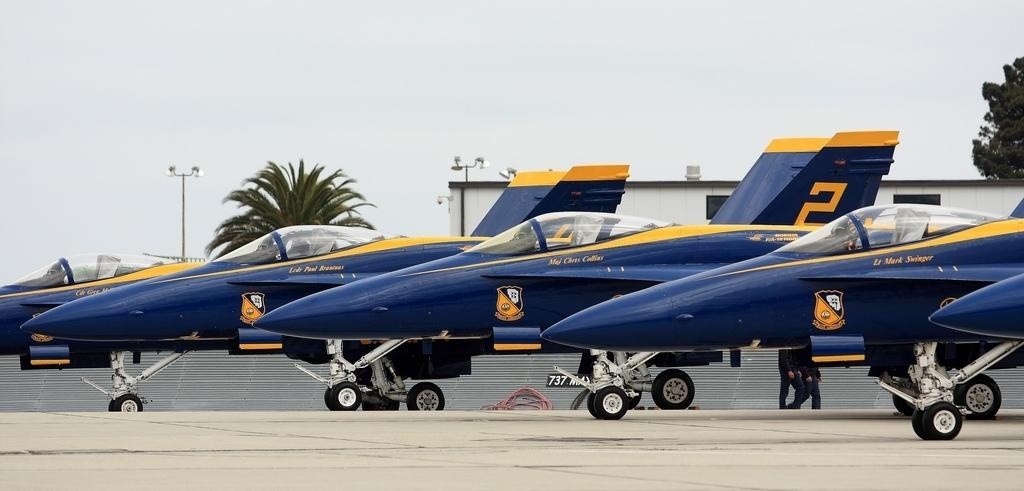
[452,155,484,234]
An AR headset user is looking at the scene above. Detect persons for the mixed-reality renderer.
[776,349,805,409]
[786,348,823,409]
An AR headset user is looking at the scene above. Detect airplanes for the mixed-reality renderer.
[927,271,1024,342]
[540,197,1024,440]
[253,130,900,412]
